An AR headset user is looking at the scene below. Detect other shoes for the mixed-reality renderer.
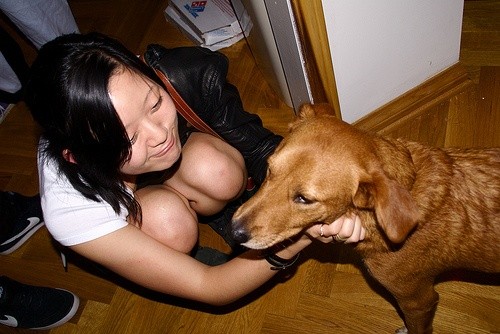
[208,199,248,252]
[190,246,231,266]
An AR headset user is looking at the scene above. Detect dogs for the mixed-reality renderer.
[230,101,500,334]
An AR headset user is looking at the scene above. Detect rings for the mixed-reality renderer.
[320,224,330,239]
[332,234,347,243]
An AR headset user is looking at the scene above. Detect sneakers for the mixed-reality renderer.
[0,190,45,256]
[0,276,79,330]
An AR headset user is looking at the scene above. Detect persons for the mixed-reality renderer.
[0,0,80,124]
[25,32,370,305]
[0,189,80,333]
